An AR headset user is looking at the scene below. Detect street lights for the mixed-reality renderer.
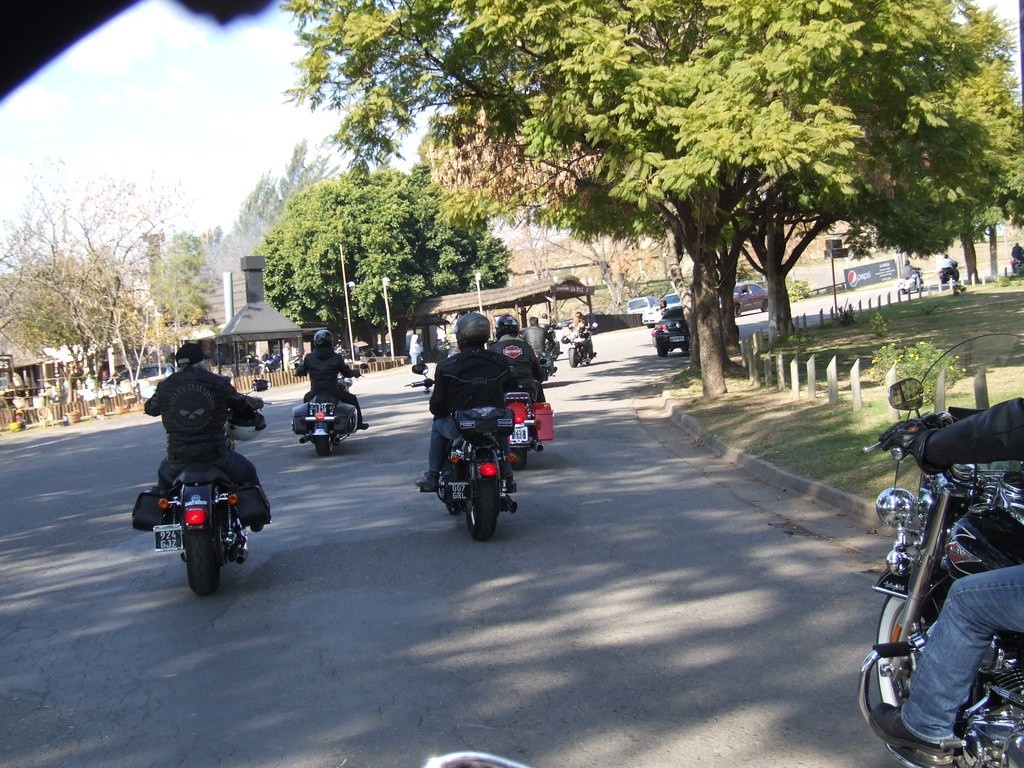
[475,272,488,351]
[382,277,395,361]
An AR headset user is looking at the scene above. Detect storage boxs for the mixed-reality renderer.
[333,402,356,433]
[534,402,554,440]
[292,402,308,434]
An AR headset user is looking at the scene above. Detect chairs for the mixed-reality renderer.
[37,407,55,428]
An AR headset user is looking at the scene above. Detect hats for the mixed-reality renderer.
[175,343,204,367]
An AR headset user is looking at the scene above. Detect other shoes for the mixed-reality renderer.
[548,367,557,376]
[356,423,369,430]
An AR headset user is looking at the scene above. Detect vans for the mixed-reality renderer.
[627,297,659,314]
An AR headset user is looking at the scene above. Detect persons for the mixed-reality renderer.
[538,312,563,354]
[143,343,267,534]
[486,314,548,404]
[409,334,421,365]
[659,300,669,315]
[569,311,597,359]
[522,317,557,376]
[295,328,370,430]
[282,341,292,371]
[940,254,958,281]
[1011,242,1024,264]
[869,396,1024,753]
[900,260,923,288]
[415,311,520,493]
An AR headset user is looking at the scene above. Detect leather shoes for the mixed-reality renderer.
[870,702,955,765]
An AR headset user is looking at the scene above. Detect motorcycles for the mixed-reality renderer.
[241,352,280,374]
[405,364,517,541]
[857,333,1024,768]
[505,392,553,471]
[561,322,600,368]
[518,326,563,382]
[289,363,369,455]
[1011,250,1024,273]
[939,262,959,284]
[898,268,924,294]
[140,379,274,597]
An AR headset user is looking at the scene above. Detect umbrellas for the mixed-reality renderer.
[410,314,450,349]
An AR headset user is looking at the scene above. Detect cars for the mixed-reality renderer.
[104,363,174,386]
[733,282,768,317]
[641,293,680,328]
[651,306,739,357]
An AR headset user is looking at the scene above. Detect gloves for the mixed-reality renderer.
[878,419,953,475]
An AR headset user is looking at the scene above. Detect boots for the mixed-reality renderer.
[505,476,517,493]
[414,470,440,490]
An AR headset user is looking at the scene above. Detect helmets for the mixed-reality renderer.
[562,336,569,344]
[495,315,519,340]
[575,311,583,319]
[313,330,332,348]
[224,412,265,440]
[542,313,548,319]
[455,313,490,344]
